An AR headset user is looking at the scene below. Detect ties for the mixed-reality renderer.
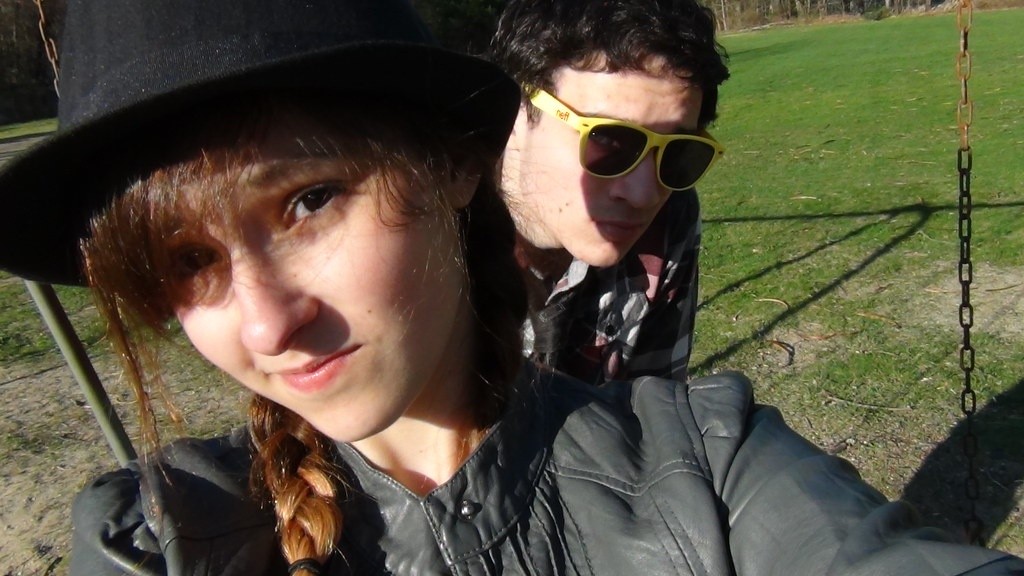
[519,270,588,373]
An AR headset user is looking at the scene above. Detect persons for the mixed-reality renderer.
[449,0,733,387]
[0,0,1024,576]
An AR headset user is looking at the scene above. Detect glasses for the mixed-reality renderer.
[519,81,728,193]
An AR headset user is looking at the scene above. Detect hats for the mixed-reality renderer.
[0,0,520,286]
